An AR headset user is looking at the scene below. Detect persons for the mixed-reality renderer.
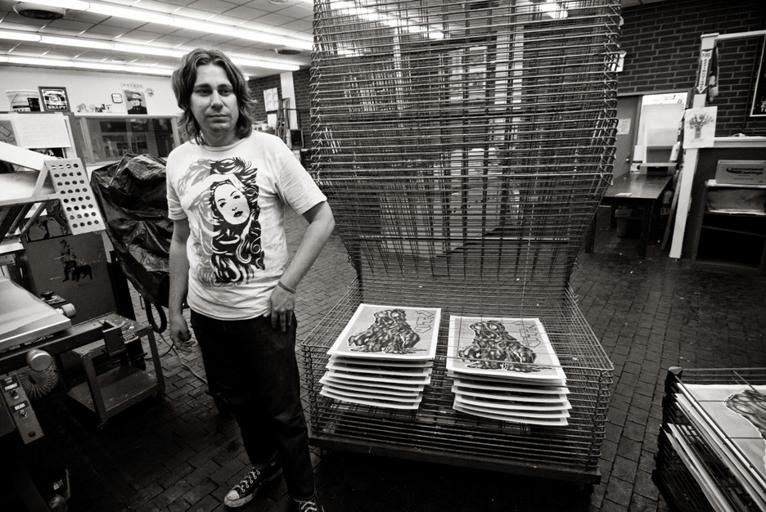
[59,240,77,283]
[127,92,147,114]
[164,47,336,512]
[182,172,265,288]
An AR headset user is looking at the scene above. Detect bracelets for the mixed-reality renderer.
[276,281,297,294]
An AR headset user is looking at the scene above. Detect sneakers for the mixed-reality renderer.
[289,474,325,512]
[224,449,283,508]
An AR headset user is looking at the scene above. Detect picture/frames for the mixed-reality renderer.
[743,31,766,121]
[38,85,71,113]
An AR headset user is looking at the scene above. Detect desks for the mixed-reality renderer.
[666,132,765,260]
[64,308,169,425]
[582,169,676,259]
